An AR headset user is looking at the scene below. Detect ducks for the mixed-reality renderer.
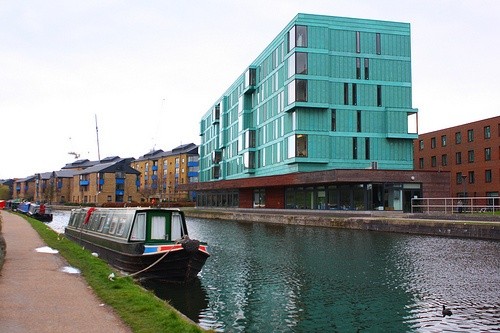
[442,303,453,317]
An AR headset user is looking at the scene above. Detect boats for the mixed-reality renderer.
[0,198,54,222]
[64,204,211,289]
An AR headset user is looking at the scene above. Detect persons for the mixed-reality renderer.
[8,201,12,208]
[39,201,46,214]
[458,200,463,213]
